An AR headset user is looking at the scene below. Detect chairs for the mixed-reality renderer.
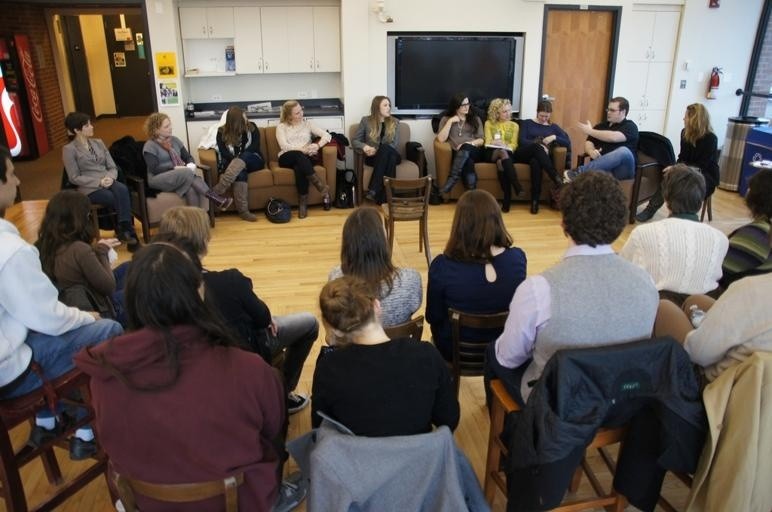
[2,359,109,511]
[700,194,715,223]
[111,140,164,244]
[381,176,431,269]
[484,337,688,512]
[384,315,424,339]
[576,130,676,223]
[112,472,245,512]
[449,308,510,396]
[91,203,112,245]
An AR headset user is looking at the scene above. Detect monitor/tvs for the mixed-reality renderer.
[394,37,516,110]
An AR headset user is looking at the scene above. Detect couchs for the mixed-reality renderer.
[350,122,428,206]
[198,126,336,213]
[434,117,567,203]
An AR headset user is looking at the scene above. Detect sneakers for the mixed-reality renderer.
[563,170,580,182]
[69,436,96,459]
[271,471,308,511]
[27,412,73,447]
[286,392,307,413]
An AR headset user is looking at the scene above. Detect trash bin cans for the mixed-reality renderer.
[718,116,769,191]
[738,125,772,197]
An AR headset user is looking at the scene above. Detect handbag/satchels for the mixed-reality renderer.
[266,197,291,223]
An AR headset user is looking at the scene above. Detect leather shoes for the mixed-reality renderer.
[363,191,378,203]
[118,227,144,253]
[553,173,562,185]
[531,201,538,214]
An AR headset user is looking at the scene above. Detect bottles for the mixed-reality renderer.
[225,46,235,72]
[186,98,194,118]
[687,304,705,328]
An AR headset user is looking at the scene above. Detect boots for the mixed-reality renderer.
[501,159,526,196]
[299,193,309,219]
[637,191,665,222]
[499,171,512,212]
[465,173,476,189]
[232,180,257,221]
[306,172,330,198]
[442,150,470,193]
[212,158,246,197]
[204,188,235,212]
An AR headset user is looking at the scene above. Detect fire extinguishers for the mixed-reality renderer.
[707,67,725,99]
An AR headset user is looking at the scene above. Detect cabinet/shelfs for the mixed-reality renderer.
[614,61,672,111]
[620,10,681,61]
[179,6,235,40]
[285,6,340,73]
[231,6,288,74]
[621,112,667,137]
